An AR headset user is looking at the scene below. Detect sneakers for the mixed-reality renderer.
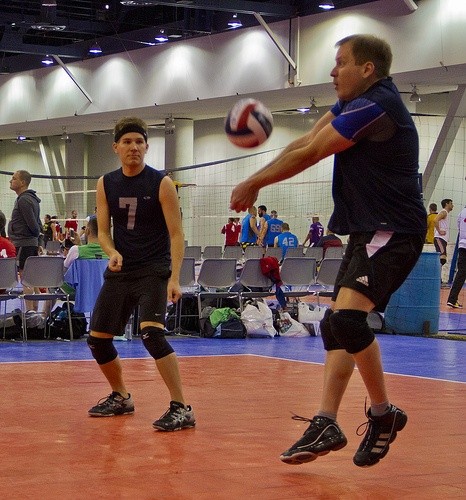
[87,392,135,416]
[447,300,463,308]
[280,416,347,465]
[352,405,407,469]
[152,400,197,430]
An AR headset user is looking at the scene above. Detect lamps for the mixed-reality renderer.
[33,0,335,65]
[408,83,423,103]
[58,124,71,142]
[19,132,39,143]
[163,112,179,131]
[302,94,320,115]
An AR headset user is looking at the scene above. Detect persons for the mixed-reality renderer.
[258,210,285,245]
[33,197,97,246]
[315,228,341,248]
[302,217,323,247]
[256,205,271,246]
[273,223,299,262]
[435,199,455,284]
[230,34,428,468]
[86,117,197,430]
[424,202,440,246]
[6,168,42,314]
[231,217,242,243]
[42,216,113,324]
[447,207,466,309]
[0,213,17,295]
[220,218,238,247]
[238,205,257,244]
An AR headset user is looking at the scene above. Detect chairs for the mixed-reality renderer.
[0,237,348,342]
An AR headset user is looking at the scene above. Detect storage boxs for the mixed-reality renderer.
[382,251,441,335]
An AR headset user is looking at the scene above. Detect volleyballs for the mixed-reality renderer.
[223,99,275,148]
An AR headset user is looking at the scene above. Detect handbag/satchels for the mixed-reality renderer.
[0,294,327,339]
